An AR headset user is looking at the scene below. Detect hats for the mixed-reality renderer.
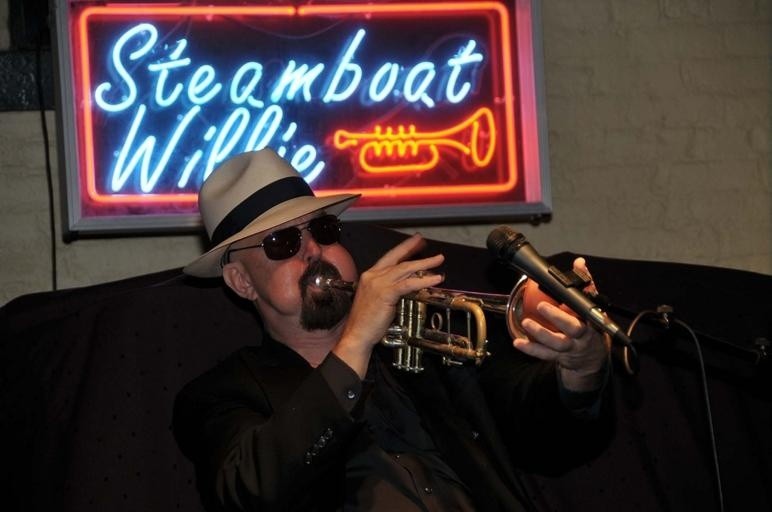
[182,147,362,278]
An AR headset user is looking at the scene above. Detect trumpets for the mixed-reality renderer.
[308,262,598,373]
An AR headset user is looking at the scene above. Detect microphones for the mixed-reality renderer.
[486,225,633,355]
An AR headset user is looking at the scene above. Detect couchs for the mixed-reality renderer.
[0,220,772,512]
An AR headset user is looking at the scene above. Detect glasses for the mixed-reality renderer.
[229,215,344,260]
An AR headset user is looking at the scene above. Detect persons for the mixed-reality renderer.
[170,145,617,511]
